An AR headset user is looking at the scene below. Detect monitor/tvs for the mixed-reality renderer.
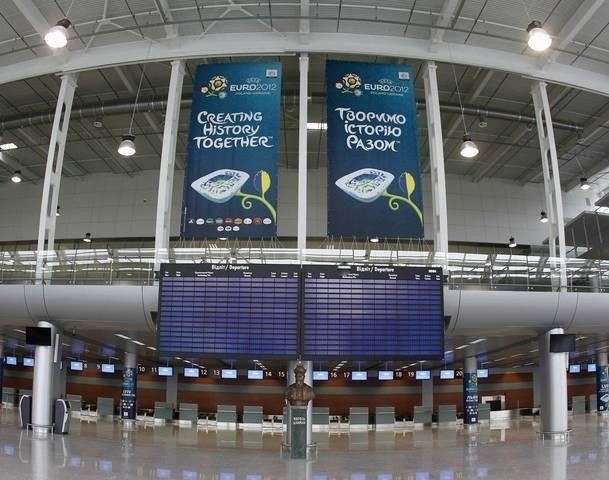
[157,366,174,377]
[22,357,35,367]
[549,334,576,353]
[476,368,489,379]
[247,369,264,380]
[183,367,200,378]
[221,368,238,380]
[312,370,329,381]
[377,370,395,381]
[26,326,51,346]
[351,370,368,381]
[101,363,115,374]
[415,369,431,380]
[70,360,84,371]
[439,369,456,380]
[5,356,18,366]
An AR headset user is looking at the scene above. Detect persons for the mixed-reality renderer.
[285,366,315,406]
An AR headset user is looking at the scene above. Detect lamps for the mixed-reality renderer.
[3,126,96,249]
[508,152,591,252]
[450,64,482,161]
[516,0,553,54]
[42,0,76,49]
[114,66,148,159]
